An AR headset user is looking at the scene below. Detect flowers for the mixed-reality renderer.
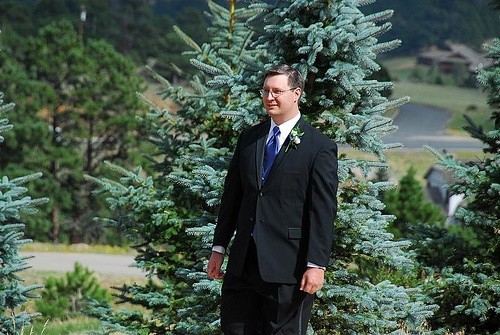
[285,123,305,152]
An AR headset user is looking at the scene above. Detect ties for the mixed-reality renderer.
[253,125,281,246]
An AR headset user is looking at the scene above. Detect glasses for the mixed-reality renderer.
[259,87,297,98]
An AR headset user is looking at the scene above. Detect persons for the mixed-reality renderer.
[207,64,339,335]
